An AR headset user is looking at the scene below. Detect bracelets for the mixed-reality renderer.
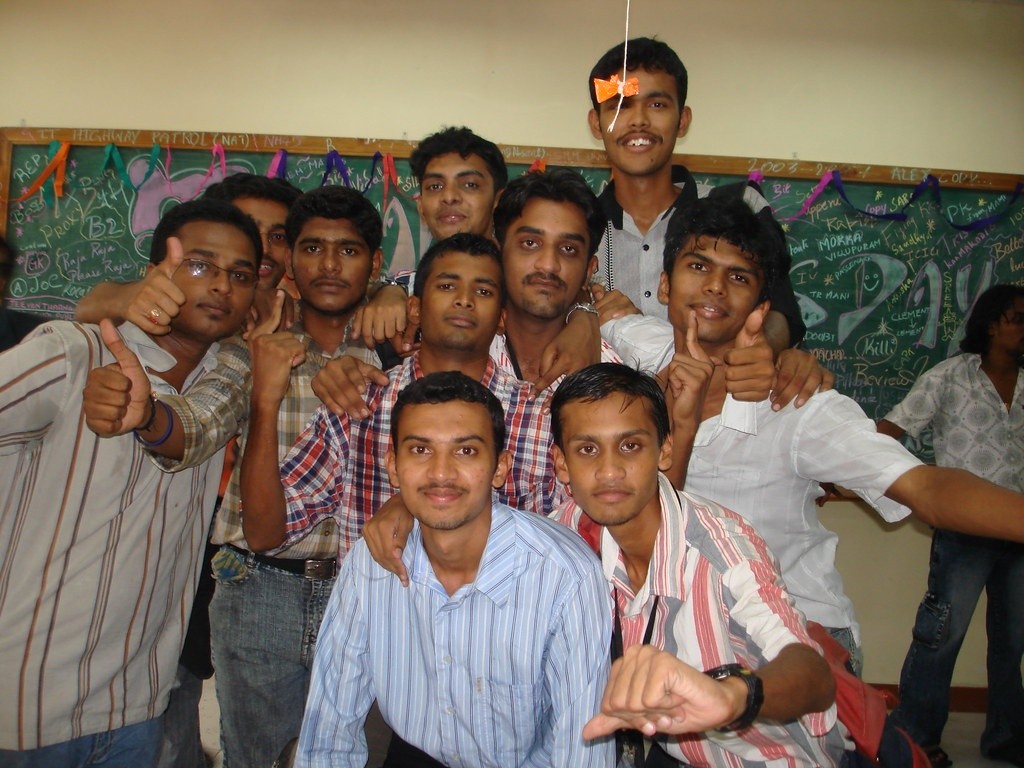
[131,395,156,432]
[134,398,172,446]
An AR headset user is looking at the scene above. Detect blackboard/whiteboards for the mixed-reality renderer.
[1,126,1023,494]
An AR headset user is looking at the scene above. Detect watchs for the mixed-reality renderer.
[565,302,599,324]
[702,664,764,733]
[371,278,409,302]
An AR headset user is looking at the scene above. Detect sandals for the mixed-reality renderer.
[990,742,1024,767]
[926,748,953,768]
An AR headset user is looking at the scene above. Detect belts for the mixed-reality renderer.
[228,542,339,581]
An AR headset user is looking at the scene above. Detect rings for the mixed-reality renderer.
[150,306,163,321]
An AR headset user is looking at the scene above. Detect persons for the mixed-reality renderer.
[0,37,1024,768]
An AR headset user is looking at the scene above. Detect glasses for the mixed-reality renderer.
[180,259,259,288]
